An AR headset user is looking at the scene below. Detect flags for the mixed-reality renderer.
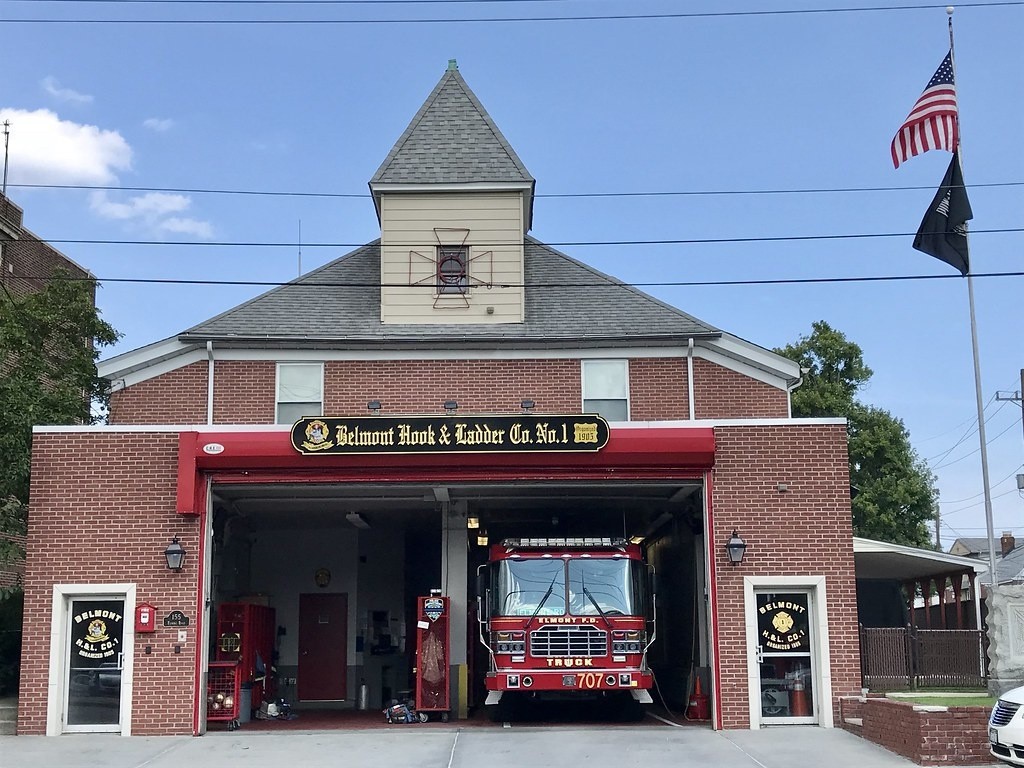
[912,151,974,278]
[890,50,959,169]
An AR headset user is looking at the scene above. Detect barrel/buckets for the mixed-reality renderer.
[240,688,252,723]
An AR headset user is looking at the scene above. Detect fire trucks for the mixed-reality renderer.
[476,535,663,721]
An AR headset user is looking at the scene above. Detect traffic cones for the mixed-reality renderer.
[791,671,809,717]
[695,676,702,695]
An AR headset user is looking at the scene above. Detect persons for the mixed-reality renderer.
[569,585,583,611]
[88,671,96,697]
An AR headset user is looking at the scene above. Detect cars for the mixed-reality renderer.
[987,684,1024,768]
[89,663,121,696]
[70,674,92,696]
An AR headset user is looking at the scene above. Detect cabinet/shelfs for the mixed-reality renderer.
[216,602,276,704]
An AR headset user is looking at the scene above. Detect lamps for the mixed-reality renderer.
[444,401,459,414]
[369,401,380,414]
[166,535,187,570]
[726,529,746,563]
[522,399,536,414]
